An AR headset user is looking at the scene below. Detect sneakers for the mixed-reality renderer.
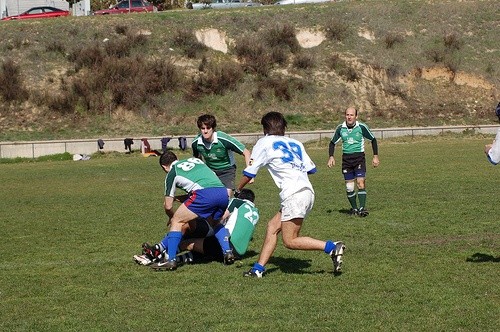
[352,209,359,215]
[243,267,263,278]
[359,207,369,217]
[143,243,160,258]
[152,259,177,271]
[330,241,346,276]
[224,252,235,265]
[133,255,151,266]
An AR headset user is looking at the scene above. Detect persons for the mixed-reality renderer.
[328,107,379,216]
[236,112,346,278]
[133,189,259,267]
[150,150,229,272]
[191,115,255,190]
[484,101,500,166]
